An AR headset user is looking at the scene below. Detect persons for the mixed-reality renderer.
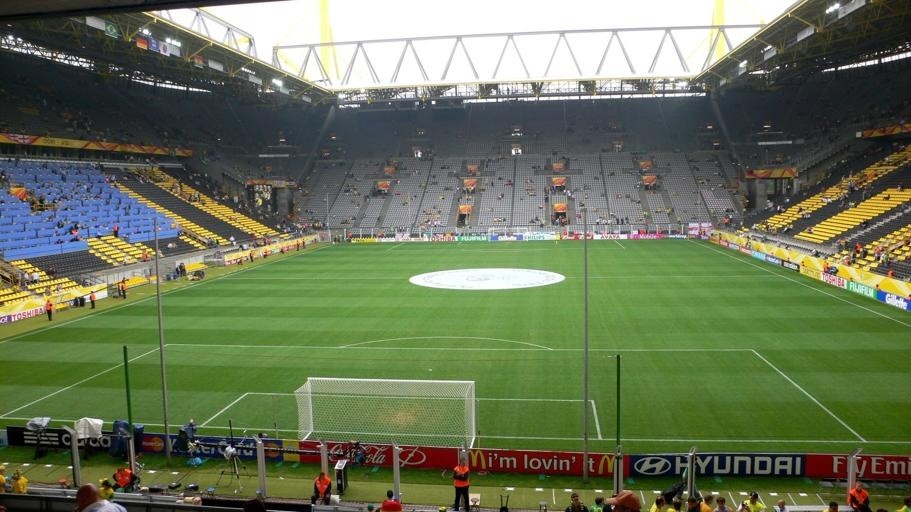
[453,457,470,510]
[566,492,590,512]
[368,503,376,511]
[0,48,292,286]
[352,442,368,469]
[112,467,141,493]
[292,126,752,234]
[10,468,28,494]
[752,99,911,302]
[90,291,96,309]
[591,481,906,512]
[181,418,198,438]
[311,471,333,506]
[98,481,115,500]
[382,490,404,512]
[1,465,12,492]
[302,238,307,250]
[297,240,301,252]
[74,484,128,512]
[46,299,54,321]
[120,280,127,300]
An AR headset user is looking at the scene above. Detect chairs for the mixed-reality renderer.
[835,223,911,282]
[0,259,84,307]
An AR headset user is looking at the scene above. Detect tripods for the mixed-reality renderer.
[214,420,252,494]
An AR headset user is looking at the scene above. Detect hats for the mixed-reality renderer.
[750,491,759,500]
[688,496,696,503]
[606,489,641,510]
